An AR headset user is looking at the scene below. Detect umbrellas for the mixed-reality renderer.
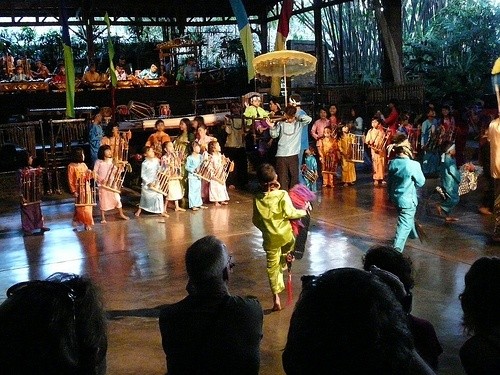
[251,49,318,107]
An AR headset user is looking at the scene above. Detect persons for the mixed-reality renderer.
[380,96,490,200]
[158,234,264,375]
[86,109,106,165]
[251,164,310,312]
[385,133,426,254]
[457,254,500,374]
[99,93,500,223]
[0,50,206,93]
[362,243,443,375]
[281,265,438,375]
[17,152,51,237]
[66,149,96,231]
[0,270,109,375]
[92,143,130,224]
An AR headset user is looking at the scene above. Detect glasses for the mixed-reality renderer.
[225,255,233,267]
[6,279,77,330]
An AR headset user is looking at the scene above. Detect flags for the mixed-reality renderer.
[231,0,258,83]
[105,14,118,87]
[62,20,76,118]
[271,0,295,96]
